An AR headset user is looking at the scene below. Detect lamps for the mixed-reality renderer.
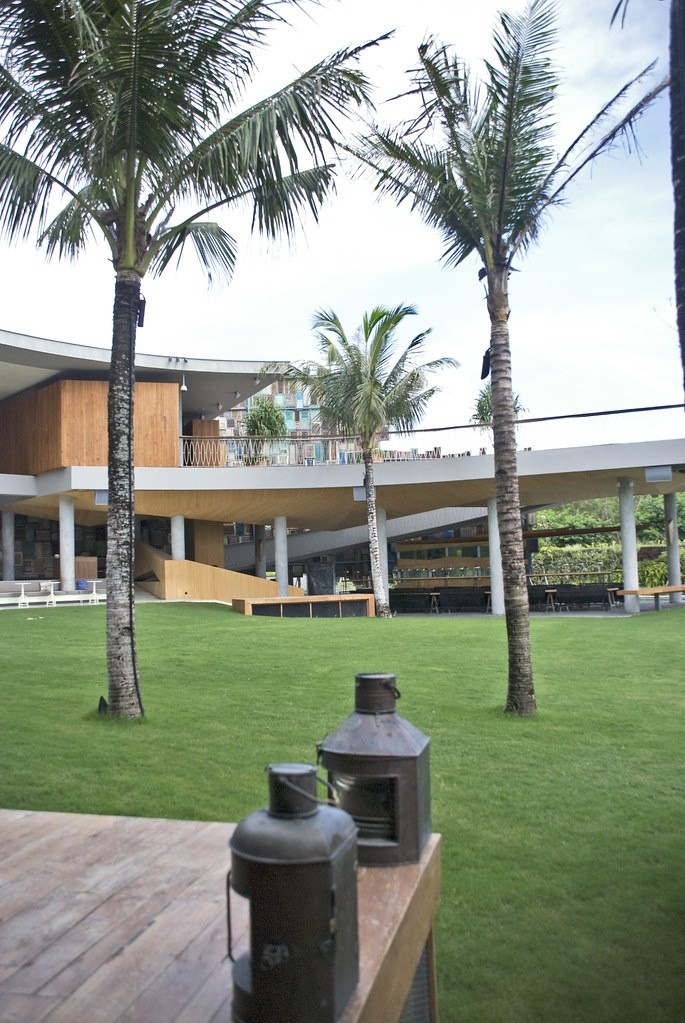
[226,761,361,1023]
[315,672,434,869]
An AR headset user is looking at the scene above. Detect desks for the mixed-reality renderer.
[52,555,97,582]
[45,581,61,608]
[0,806,443,1023]
[15,582,31,609]
[86,580,103,605]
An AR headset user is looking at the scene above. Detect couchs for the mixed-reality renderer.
[0,578,108,605]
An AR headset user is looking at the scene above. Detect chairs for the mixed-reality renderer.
[351,582,625,613]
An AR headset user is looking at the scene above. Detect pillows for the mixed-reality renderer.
[39,581,52,593]
[76,579,88,591]
